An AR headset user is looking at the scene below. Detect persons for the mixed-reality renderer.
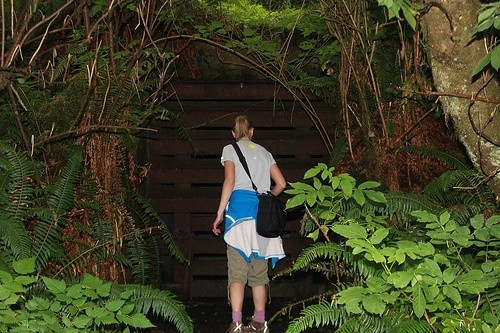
[211,114,287,333]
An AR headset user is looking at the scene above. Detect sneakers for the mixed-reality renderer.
[228,320,243,333]
[242,318,271,333]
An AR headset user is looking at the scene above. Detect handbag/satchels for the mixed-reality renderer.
[257,193,288,239]
